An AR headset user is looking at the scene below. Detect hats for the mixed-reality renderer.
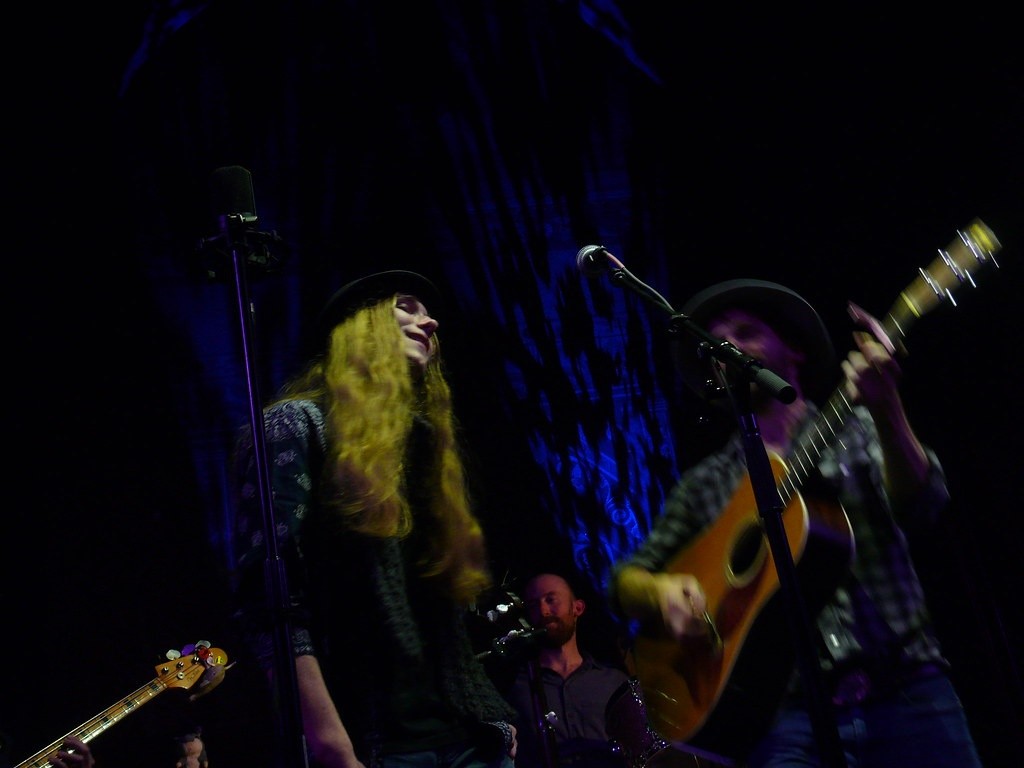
[308,248,444,351]
[679,278,835,386]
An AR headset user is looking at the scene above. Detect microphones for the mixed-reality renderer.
[577,245,609,276]
[212,164,259,326]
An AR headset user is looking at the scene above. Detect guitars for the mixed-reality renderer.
[12,639,227,768]
[627,218,1003,749]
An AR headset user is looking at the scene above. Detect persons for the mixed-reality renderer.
[612,280,984,767]
[230,267,519,768]
[499,574,631,768]
[172,721,210,768]
[47,738,95,768]
